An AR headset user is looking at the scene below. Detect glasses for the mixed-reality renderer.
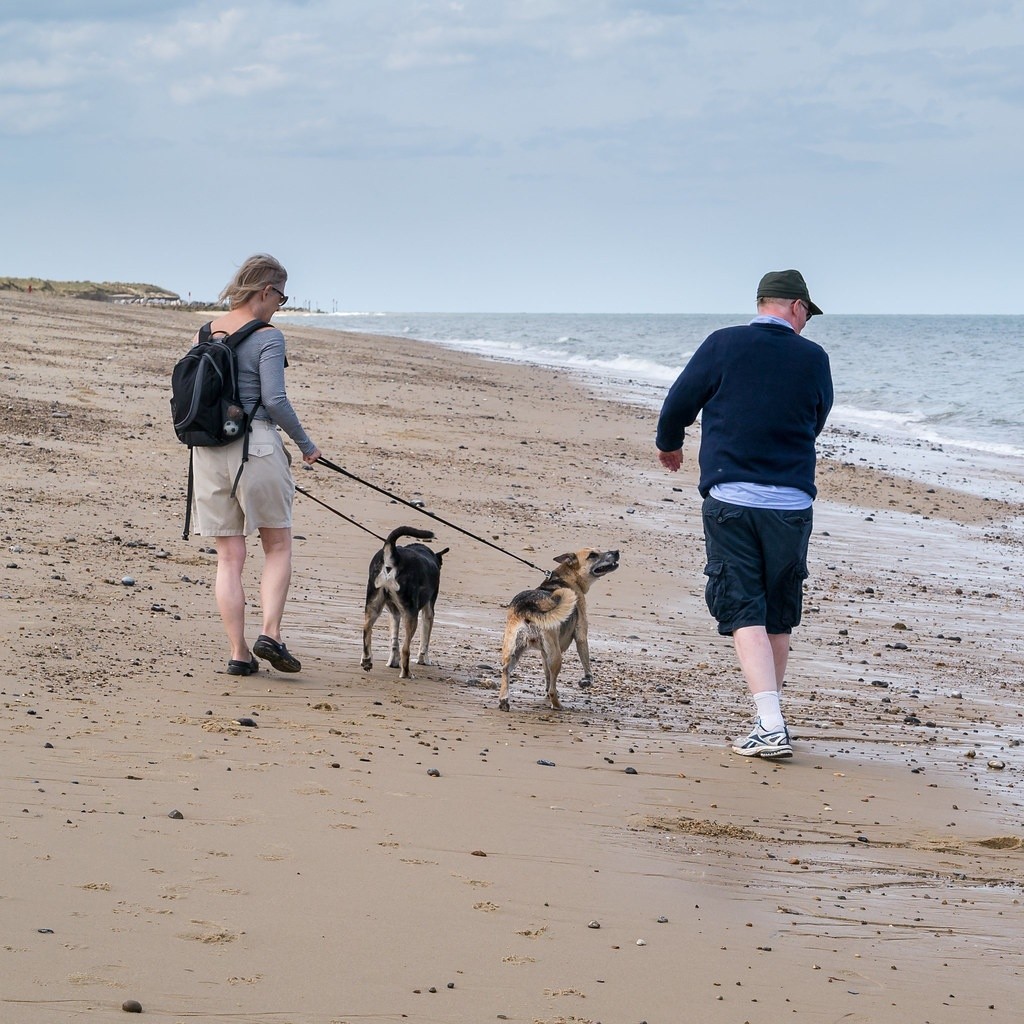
[271,287,288,306]
[791,300,812,321]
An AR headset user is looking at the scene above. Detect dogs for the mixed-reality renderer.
[361,526,451,679]
[497,548,621,712]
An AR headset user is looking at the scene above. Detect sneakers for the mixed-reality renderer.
[731,719,793,758]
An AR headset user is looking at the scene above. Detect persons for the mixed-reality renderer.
[192,253,321,676]
[656,270,834,759]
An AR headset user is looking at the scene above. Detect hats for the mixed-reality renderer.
[756,270,823,315]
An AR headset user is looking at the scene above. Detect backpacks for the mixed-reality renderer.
[170,318,288,449]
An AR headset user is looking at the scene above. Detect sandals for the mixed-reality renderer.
[227,652,259,676]
[253,635,301,673]
